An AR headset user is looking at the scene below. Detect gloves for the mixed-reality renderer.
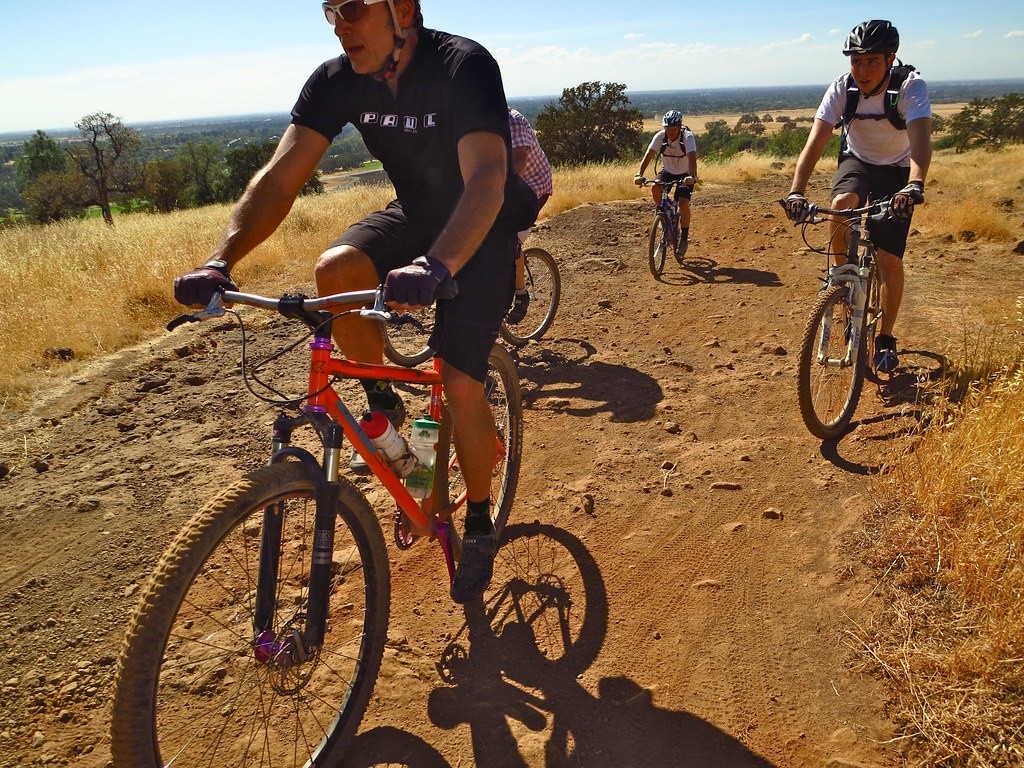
[684,176,695,186]
[785,194,807,223]
[634,175,643,188]
[888,180,924,219]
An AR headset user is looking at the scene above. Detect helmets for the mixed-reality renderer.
[662,110,683,127]
[843,19,899,56]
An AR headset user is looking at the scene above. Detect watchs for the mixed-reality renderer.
[690,175,696,180]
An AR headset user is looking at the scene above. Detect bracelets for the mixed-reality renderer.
[634,175,641,177]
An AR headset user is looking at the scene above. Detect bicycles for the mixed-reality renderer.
[378,226,562,368]
[773,194,924,439]
[638,178,703,276]
[106,274,525,768]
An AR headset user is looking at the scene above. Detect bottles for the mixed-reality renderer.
[859,257,872,299]
[359,411,415,477]
[404,412,439,498]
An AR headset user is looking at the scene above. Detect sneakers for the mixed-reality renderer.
[450,521,499,604]
[817,263,837,295]
[348,390,406,475]
[875,334,899,371]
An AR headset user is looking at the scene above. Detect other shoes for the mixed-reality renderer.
[507,290,529,325]
[678,240,688,256]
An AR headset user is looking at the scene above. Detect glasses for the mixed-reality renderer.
[322,0,387,25]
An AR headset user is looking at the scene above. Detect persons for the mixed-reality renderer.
[634,110,697,255]
[174,0,518,604]
[506,102,553,325]
[784,20,933,373]
